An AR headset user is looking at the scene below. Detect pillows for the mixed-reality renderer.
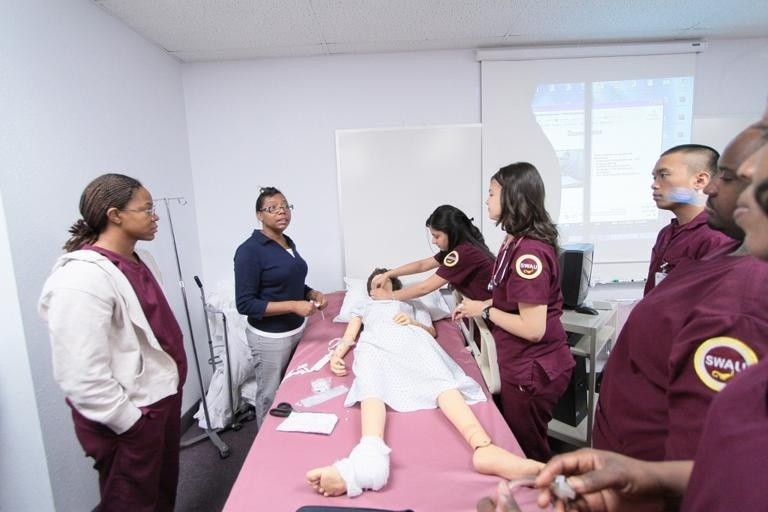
[332,276,451,323]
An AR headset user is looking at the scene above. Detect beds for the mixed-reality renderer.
[221,290,555,512]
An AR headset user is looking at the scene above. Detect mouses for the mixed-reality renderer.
[577,307,598,315]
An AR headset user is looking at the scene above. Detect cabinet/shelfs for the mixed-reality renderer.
[547,295,619,448]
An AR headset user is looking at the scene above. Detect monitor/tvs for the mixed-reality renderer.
[561,242,595,310]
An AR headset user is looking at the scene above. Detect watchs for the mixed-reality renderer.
[482,306,493,321]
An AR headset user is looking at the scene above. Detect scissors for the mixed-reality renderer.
[269,403,323,417]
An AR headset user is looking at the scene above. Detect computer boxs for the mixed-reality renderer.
[553,355,588,427]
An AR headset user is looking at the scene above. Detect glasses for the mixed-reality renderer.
[119,207,155,215]
[258,205,292,213]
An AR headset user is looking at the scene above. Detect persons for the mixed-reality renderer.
[39,173,187,512]
[643,144,741,298]
[234,187,328,431]
[477,131,767,512]
[451,163,576,463]
[592,122,767,465]
[370,205,497,349]
[306,267,546,498]
